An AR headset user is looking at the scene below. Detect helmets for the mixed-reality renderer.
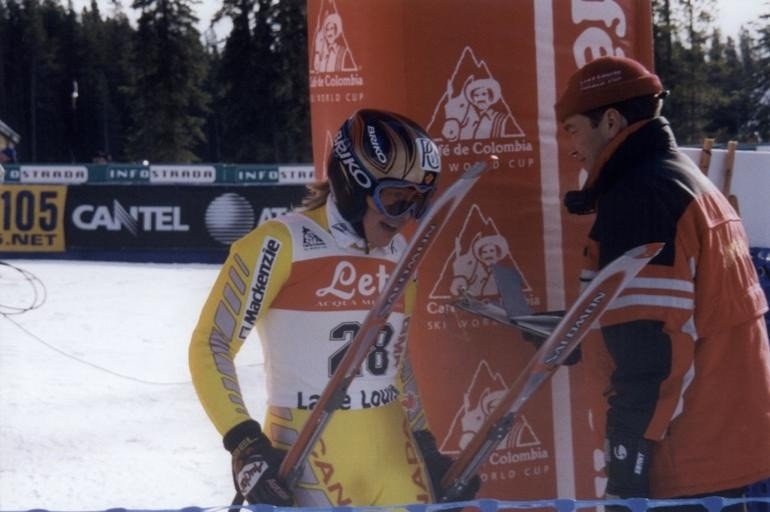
[326,108,440,223]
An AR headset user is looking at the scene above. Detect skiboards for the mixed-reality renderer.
[277,163,666,501]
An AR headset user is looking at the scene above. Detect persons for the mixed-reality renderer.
[521,56,770,510]
[188,109,481,510]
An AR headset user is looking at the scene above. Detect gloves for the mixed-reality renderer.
[220,417,298,509]
[414,433,483,506]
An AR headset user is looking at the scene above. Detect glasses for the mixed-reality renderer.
[358,176,438,222]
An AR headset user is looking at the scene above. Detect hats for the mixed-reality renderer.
[554,56,666,122]
